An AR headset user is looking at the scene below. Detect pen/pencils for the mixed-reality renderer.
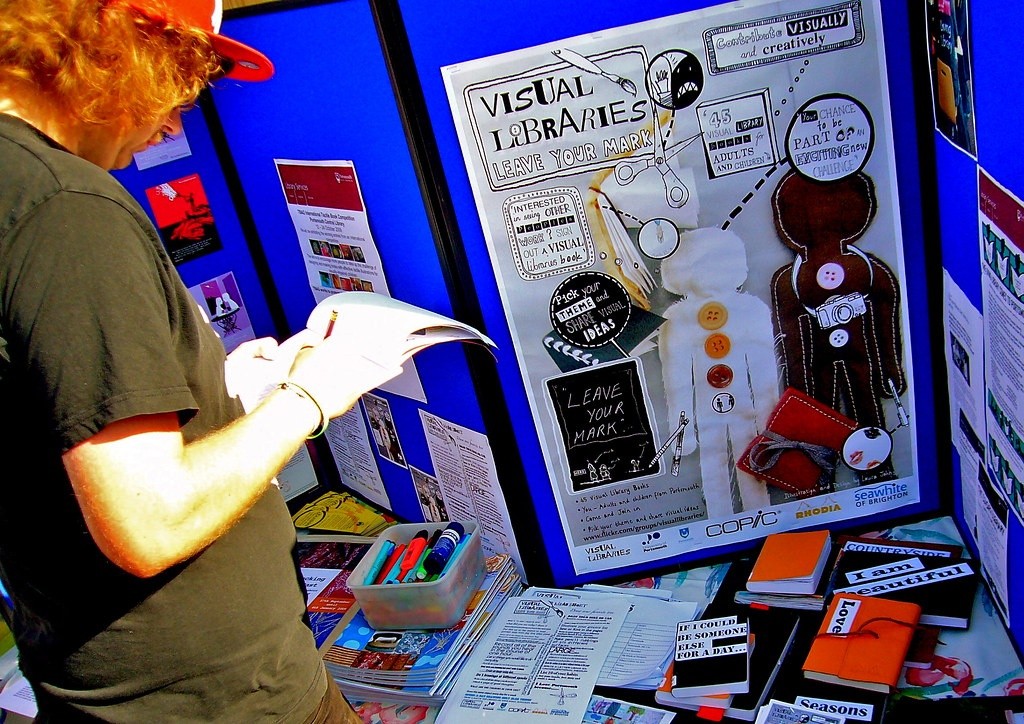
[324,310,337,339]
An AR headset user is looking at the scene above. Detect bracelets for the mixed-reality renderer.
[276,381,329,440]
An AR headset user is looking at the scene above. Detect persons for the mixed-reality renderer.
[0,0,363,724]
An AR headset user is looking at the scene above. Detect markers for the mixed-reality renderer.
[363,529,474,585]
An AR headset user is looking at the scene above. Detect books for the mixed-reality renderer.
[222,290,981,724]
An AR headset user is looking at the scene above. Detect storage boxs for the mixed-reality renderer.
[346,522,490,630]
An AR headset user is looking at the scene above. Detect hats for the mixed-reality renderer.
[125,0,276,82]
